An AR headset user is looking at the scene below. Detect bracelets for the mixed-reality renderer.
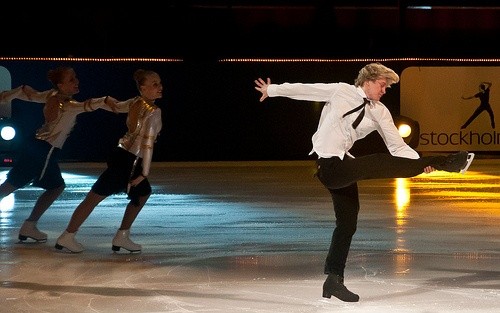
[20,84,26,91]
[141,174,147,179]
[103,95,108,103]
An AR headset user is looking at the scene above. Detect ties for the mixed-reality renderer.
[342,97,370,129]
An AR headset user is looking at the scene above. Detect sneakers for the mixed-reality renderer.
[322,275,359,303]
[17,219,48,242]
[54,229,84,252]
[111,229,142,255]
[443,151,474,174]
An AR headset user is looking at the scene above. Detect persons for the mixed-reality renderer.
[255,62,474,303]
[0,68,163,252]
